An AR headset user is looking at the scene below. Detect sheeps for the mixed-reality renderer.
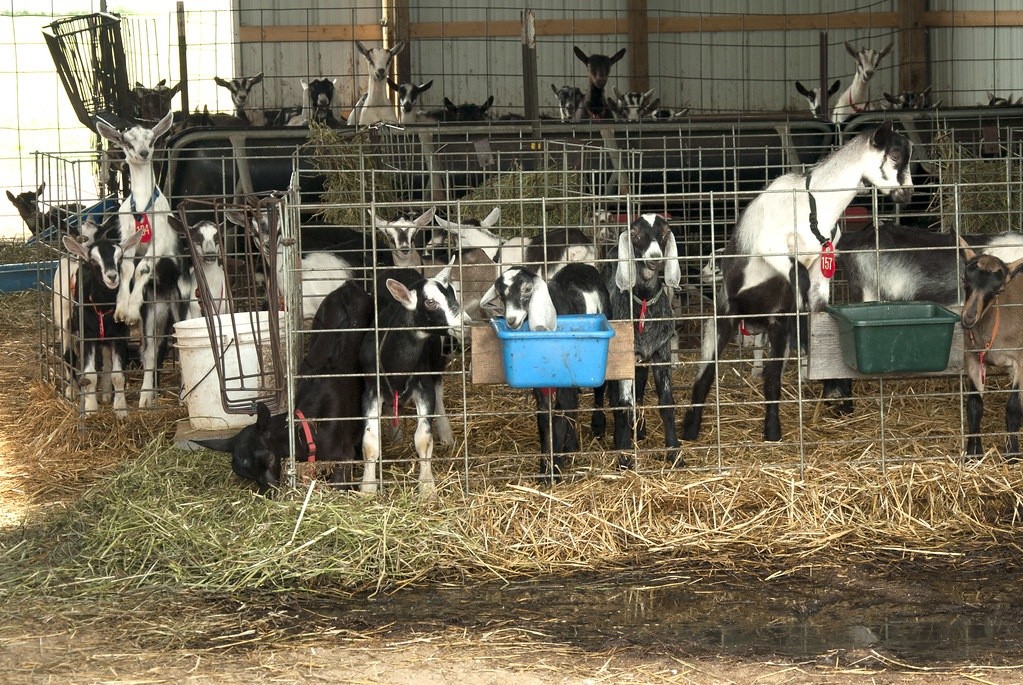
[5,40,1023,496]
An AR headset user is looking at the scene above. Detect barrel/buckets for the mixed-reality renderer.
[172,309,288,429]
